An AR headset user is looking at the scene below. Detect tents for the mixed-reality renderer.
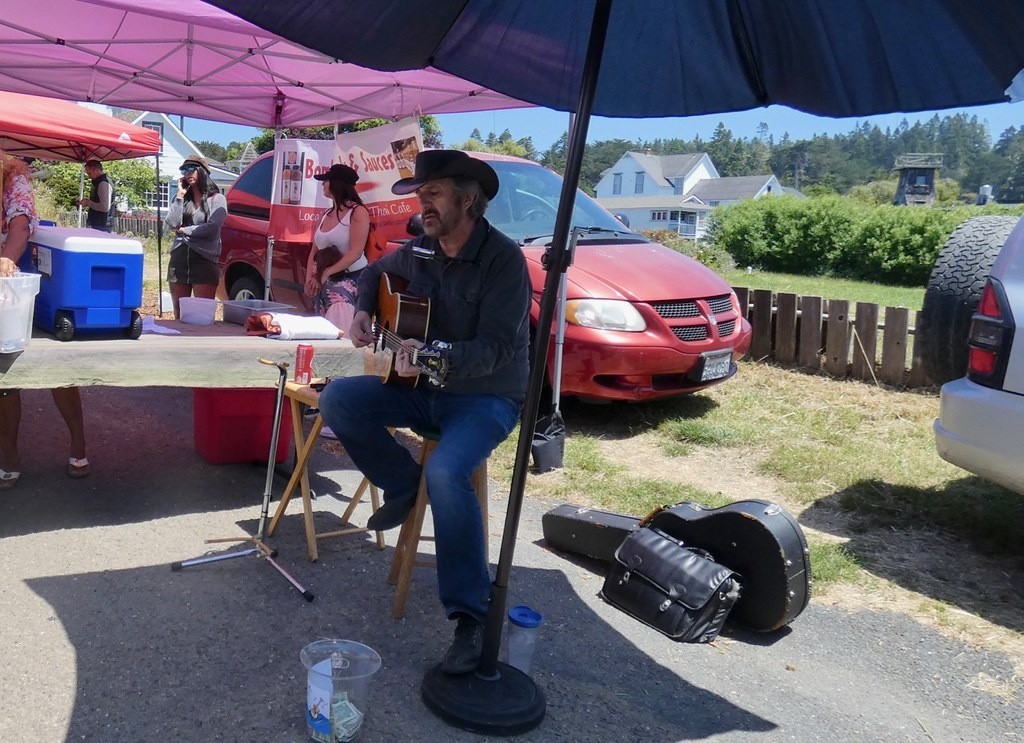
[0,0,575,416]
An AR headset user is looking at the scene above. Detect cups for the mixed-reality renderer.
[300,638,382,743]
[507,605,542,674]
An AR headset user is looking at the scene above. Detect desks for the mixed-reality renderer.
[265,374,389,565]
[0,316,366,501]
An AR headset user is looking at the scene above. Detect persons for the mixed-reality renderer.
[167,156,228,320]
[304,164,370,316]
[77,159,115,232]
[0,149,40,273]
[316,248,360,341]
[318,148,532,685]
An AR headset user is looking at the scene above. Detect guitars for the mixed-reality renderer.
[365,275,455,390]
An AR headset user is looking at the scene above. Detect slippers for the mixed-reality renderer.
[0,468,20,490]
[67,454,91,477]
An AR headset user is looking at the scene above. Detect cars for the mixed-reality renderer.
[217,147,753,406]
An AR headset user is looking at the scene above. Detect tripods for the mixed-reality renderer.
[169,358,315,603]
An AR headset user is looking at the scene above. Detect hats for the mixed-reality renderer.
[180,154,211,175]
[313,165,360,187]
[390,150,499,200]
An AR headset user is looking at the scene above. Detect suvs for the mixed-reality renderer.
[915,212,1024,497]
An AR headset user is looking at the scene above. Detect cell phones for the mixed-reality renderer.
[183,179,188,187]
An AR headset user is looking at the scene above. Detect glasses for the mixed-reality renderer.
[181,166,198,175]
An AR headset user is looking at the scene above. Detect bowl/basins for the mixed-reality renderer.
[179,297,217,325]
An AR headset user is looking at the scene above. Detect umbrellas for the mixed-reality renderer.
[203,0,1024,680]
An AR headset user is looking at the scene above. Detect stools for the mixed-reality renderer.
[384,424,493,623]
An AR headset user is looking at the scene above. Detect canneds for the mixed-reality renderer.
[293,344,314,385]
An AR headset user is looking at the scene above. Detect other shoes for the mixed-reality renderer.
[367,489,417,530]
[440,618,483,672]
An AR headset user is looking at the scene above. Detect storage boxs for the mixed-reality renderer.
[0,271,42,351]
[189,386,295,466]
[177,295,218,328]
[222,298,297,329]
